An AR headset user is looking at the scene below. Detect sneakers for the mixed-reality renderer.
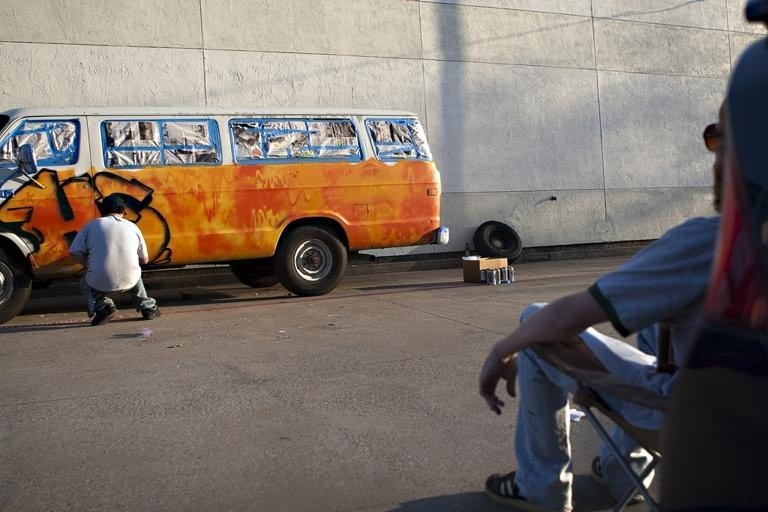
[485,469,573,512]
[141,305,161,320]
[90,302,116,326]
[591,455,645,502]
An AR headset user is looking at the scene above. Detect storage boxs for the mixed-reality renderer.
[463,258,508,283]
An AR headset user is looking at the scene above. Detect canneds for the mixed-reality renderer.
[480,266,515,285]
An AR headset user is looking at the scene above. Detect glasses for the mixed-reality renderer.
[703,122,723,152]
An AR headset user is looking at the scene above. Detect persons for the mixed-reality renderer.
[477,116,724,512]
[645,36,768,511]
[69,193,160,328]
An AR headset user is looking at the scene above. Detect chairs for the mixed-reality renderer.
[531,313,702,512]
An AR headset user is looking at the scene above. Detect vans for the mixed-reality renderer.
[0,107,449,325]
